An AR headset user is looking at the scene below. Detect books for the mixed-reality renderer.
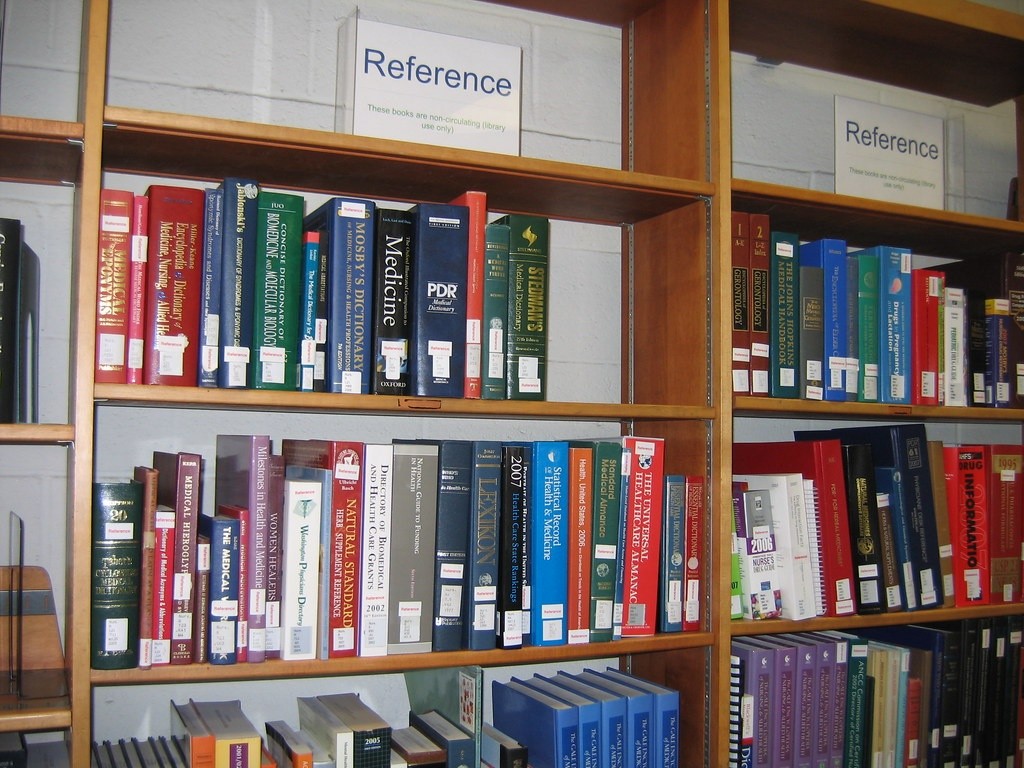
[731,422,1024,621]
[92,433,708,670]
[733,213,1024,410]
[730,614,1024,768]
[92,667,680,768]
[99,182,553,400]
[0,219,37,424]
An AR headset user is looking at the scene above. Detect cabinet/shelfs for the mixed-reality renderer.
[0,0,1024,768]
